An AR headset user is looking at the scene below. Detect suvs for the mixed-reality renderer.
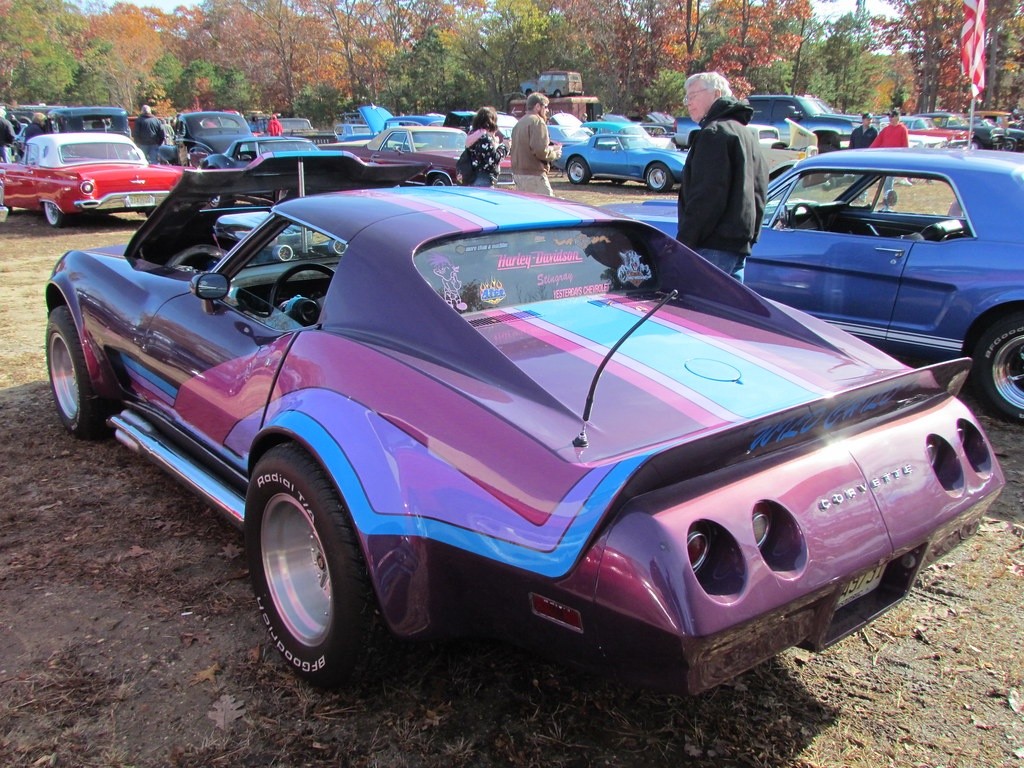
[245,117,338,147]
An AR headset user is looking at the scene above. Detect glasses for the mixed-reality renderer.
[540,104,548,110]
[682,88,710,106]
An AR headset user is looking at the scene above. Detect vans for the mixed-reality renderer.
[521,71,584,99]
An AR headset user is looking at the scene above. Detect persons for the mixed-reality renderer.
[510,92,562,196]
[868,110,909,194]
[267,114,283,137]
[465,106,511,187]
[676,72,770,285]
[24,112,47,146]
[0,108,23,164]
[848,113,878,204]
[134,105,177,165]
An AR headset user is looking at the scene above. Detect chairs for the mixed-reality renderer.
[897,219,962,240]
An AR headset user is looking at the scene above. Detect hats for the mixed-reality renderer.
[886,110,900,117]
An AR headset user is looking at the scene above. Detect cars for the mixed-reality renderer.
[314,126,516,191]
[0,132,197,227]
[46,106,134,159]
[594,147,1024,428]
[170,111,256,171]
[199,136,324,171]
[334,93,1024,195]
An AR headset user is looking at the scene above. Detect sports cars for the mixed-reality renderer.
[44,147,1006,697]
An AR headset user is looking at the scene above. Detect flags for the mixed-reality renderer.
[960,0,985,98]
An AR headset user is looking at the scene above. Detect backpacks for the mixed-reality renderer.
[454,139,485,186]
